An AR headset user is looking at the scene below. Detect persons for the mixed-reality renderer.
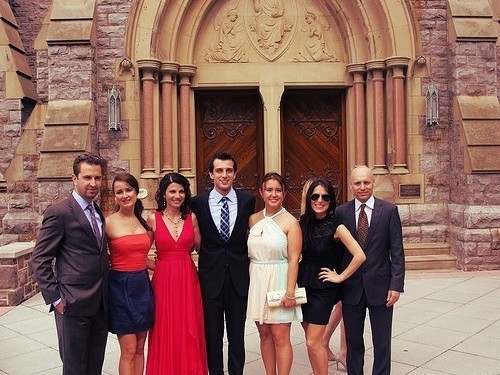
[301,180,348,372]
[332,166,405,375]
[144,172,209,375]
[154,152,257,375]
[104,172,155,375]
[31,153,108,375]
[297,179,366,375]
[248,171,302,375]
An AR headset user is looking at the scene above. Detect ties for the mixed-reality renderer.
[86,203,102,250]
[219,197,230,243]
[357,204,369,253]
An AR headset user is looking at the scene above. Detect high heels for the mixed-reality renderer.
[328,354,337,361]
[336,354,347,372]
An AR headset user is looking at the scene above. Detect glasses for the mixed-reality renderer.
[77,153,101,161]
[309,193,332,202]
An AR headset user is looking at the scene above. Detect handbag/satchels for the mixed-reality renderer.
[266,287,307,307]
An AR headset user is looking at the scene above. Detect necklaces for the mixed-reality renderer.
[119,210,139,227]
[163,210,184,239]
